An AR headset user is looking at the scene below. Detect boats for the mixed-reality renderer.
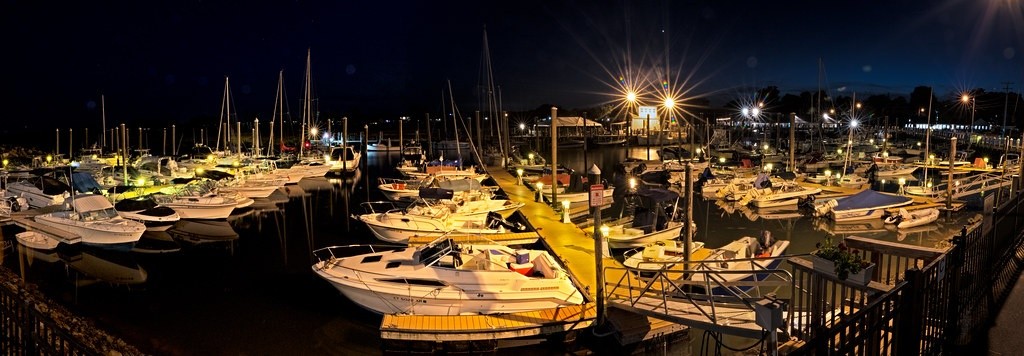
[0,21,1023,356]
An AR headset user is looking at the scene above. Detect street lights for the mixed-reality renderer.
[664,97,675,131]
[626,91,636,113]
